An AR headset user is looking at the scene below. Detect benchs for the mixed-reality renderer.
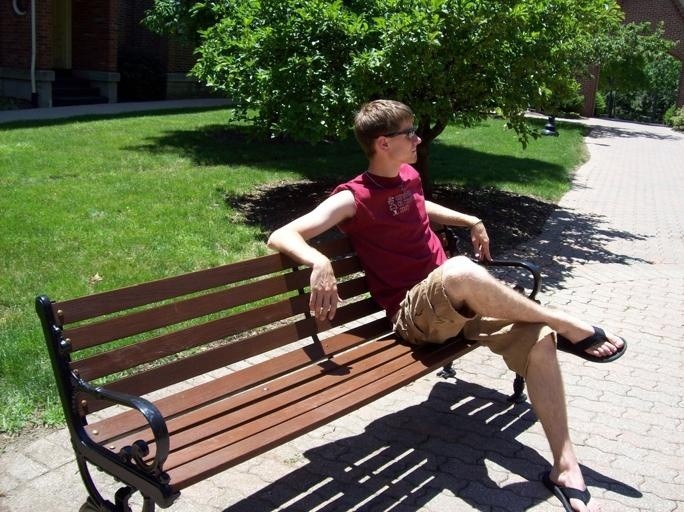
[35,232,540,511]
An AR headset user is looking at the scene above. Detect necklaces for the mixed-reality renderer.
[364,172,405,189]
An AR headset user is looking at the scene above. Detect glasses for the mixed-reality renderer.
[385,129,416,139]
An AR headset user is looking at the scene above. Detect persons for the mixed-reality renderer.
[269,98,626,512]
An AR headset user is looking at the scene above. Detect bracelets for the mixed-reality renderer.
[470,219,484,226]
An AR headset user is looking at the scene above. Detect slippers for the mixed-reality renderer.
[542,472,590,511]
[557,325,627,363]
